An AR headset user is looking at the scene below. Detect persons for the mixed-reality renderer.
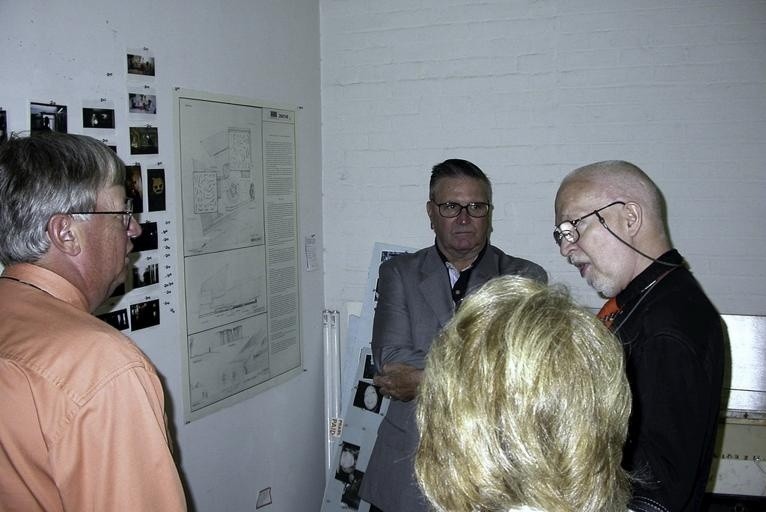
[552,159,734,512]
[39,116,52,132]
[338,447,356,476]
[0,133,194,512]
[362,383,378,411]
[131,96,153,112]
[408,269,639,512]
[353,156,548,512]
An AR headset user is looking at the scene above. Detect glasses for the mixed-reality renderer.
[553,201,626,248]
[430,199,490,218]
[44,198,134,232]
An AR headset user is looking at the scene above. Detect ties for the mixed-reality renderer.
[596,296,623,329]
